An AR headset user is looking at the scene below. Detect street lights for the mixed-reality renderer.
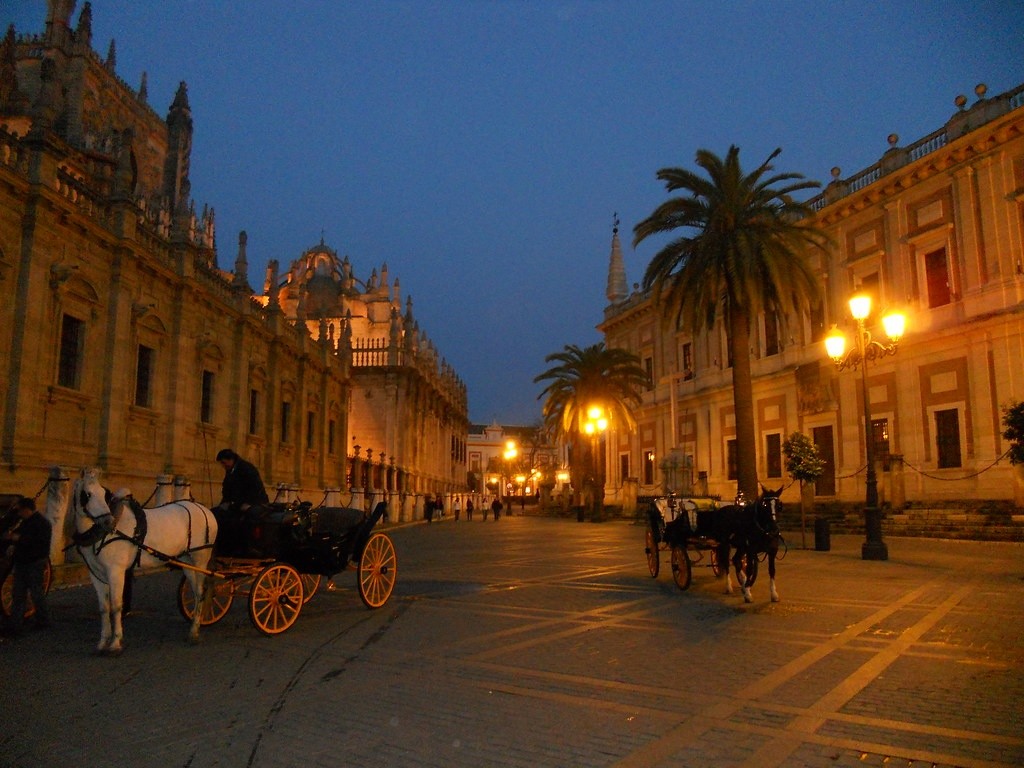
[823,292,911,562]
[586,405,612,524]
[506,440,516,515]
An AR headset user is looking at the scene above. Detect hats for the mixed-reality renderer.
[19,497,36,510]
[216,449,235,461]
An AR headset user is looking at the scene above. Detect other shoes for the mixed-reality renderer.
[1,623,25,634]
[26,616,51,631]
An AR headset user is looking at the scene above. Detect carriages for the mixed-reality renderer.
[645,483,788,603]
[66,466,397,656]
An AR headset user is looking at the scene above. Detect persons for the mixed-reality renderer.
[466,498,474,521]
[0,494,52,635]
[435,496,444,520]
[492,499,503,519]
[454,498,461,522]
[216,449,268,510]
[426,496,433,524]
[482,498,490,521]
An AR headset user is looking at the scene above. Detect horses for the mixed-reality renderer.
[68,465,220,656]
[708,481,785,604]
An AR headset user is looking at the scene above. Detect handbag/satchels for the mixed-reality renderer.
[497,502,503,510]
